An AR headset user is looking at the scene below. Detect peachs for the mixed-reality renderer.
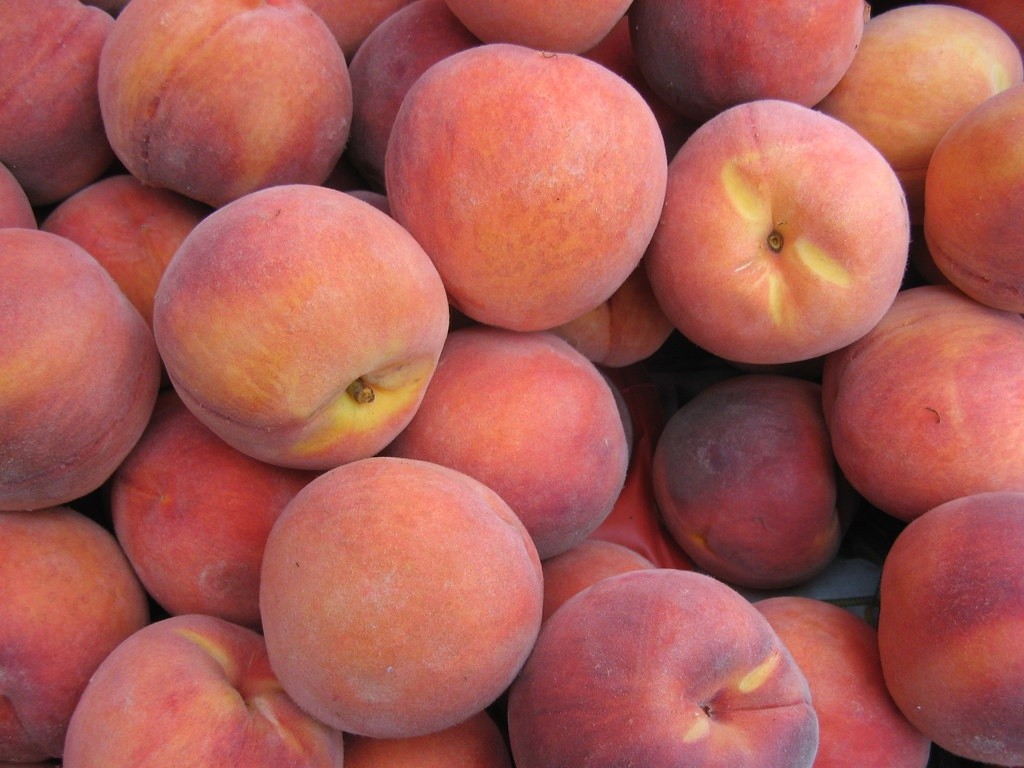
[0,0,1024,768]
